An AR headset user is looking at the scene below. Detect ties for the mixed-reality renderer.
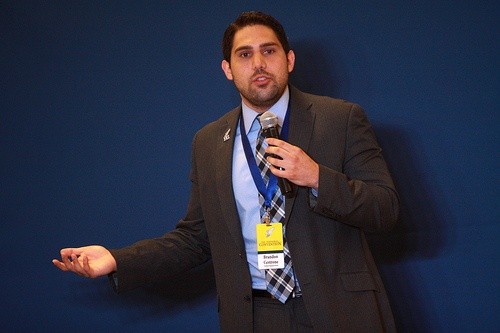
[256,114,296,306]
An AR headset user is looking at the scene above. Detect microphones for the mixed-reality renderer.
[260,111,293,198]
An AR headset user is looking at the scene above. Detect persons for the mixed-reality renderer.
[52,10,401,333]
